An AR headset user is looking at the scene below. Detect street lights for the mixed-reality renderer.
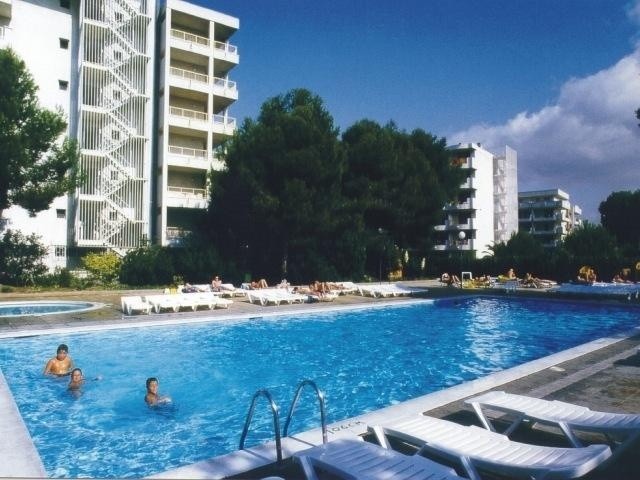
[459,231,466,273]
[378,228,384,285]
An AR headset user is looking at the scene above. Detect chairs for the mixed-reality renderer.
[480,272,640,297]
[120,277,429,317]
[370,411,612,479]
[293,435,472,480]
[465,390,640,470]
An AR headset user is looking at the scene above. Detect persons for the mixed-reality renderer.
[67,368,84,400]
[44,343,74,376]
[475,264,638,288]
[211,276,232,291]
[251,278,268,289]
[144,377,172,410]
[294,280,347,297]
[182,282,203,293]
